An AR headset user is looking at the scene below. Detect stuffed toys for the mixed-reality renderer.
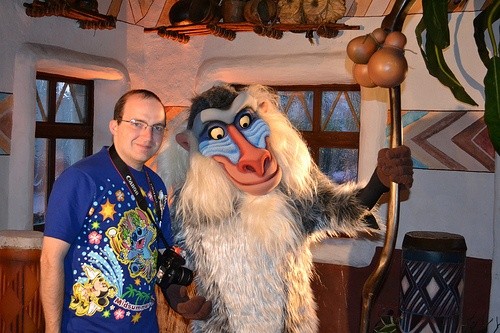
[158,81,415,333]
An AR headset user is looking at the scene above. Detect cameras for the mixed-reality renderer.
[156,249,194,289]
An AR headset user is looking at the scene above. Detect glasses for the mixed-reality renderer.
[116,118,168,136]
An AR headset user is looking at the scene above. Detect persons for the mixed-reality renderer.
[38,88,176,333]
